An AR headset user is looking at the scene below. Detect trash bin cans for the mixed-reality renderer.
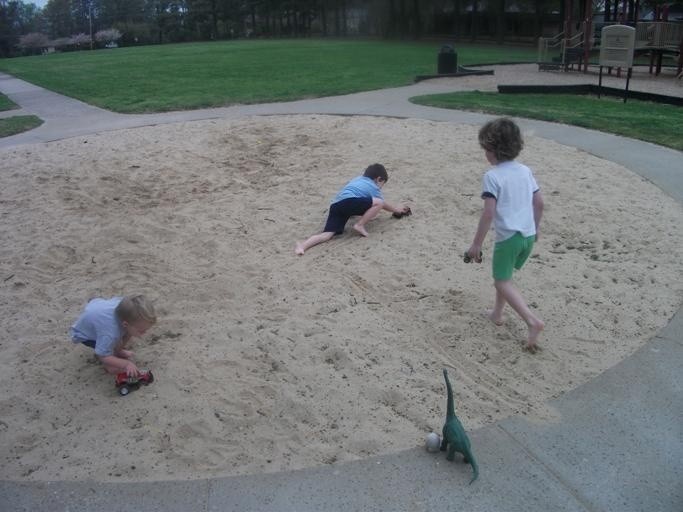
[438,44,457,74]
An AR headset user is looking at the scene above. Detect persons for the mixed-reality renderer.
[296,163,409,255]
[470,117,545,350]
[71,293,157,378]
